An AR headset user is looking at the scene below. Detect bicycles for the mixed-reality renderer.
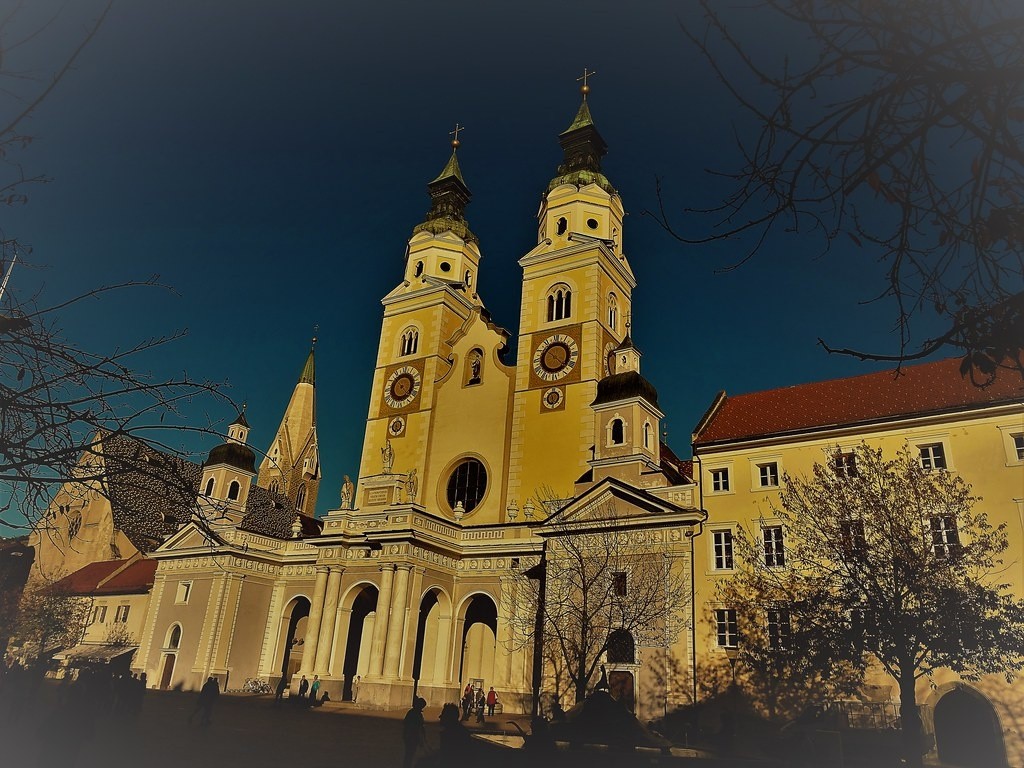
[243,677,273,695]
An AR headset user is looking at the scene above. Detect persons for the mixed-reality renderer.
[56,667,146,717]
[299,675,309,700]
[381,440,395,473]
[341,476,354,509]
[351,676,360,703]
[439,703,470,768]
[405,469,418,500]
[403,698,426,768]
[508,717,557,752]
[191,677,219,726]
[460,683,485,723]
[550,704,564,720]
[487,687,498,716]
[7,646,13,667]
[310,675,319,708]
[275,676,287,700]
[468,354,481,384]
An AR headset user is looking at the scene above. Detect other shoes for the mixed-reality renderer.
[492,714,494,716]
[460,712,483,724]
[488,714,490,716]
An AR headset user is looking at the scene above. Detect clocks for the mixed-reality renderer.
[533,334,577,382]
[384,365,421,408]
[542,386,564,410]
[603,340,618,378]
[389,415,406,437]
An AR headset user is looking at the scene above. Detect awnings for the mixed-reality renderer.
[53,647,135,664]
